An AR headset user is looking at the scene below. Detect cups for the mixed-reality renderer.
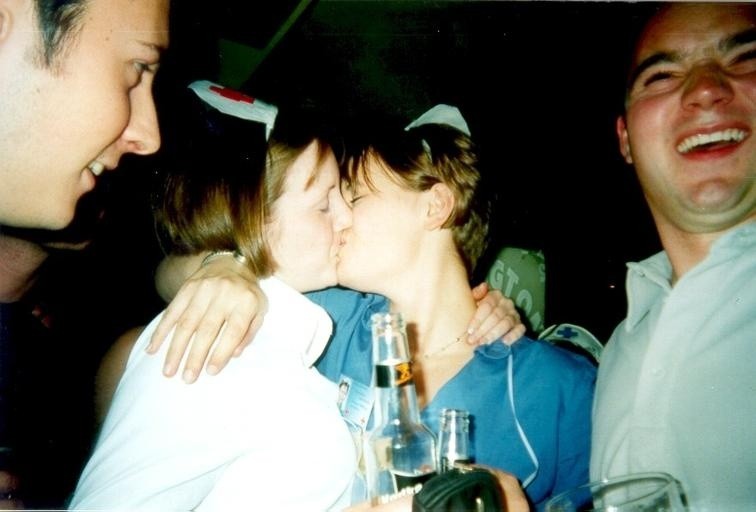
[545,472,685,512]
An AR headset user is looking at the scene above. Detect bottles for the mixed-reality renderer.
[359,312,478,512]
